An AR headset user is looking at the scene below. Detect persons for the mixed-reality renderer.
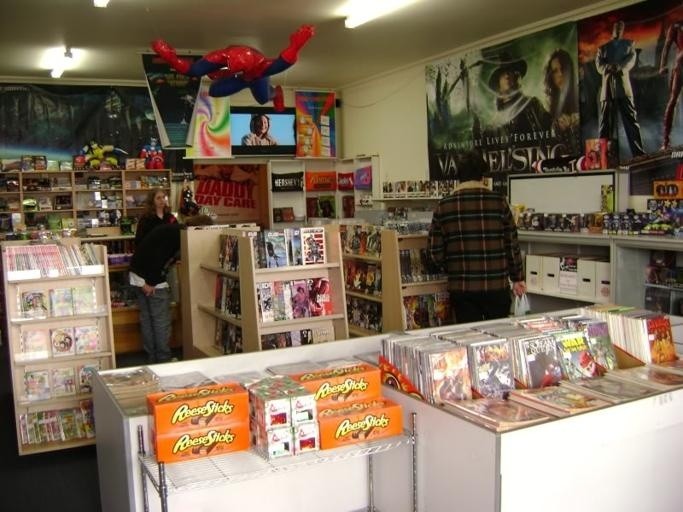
[128,216,213,363]
[473,56,552,148]
[595,19,646,165]
[133,187,178,252]
[657,12,682,153]
[426,154,527,323]
[240,114,278,146]
[382,304,683,434]
[546,48,580,143]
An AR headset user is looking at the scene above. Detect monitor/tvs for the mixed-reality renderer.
[230,106,296,157]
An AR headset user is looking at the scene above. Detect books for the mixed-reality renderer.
[5,240,100,445]
[212,228,337,356]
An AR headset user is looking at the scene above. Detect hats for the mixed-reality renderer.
[488,47,527,88]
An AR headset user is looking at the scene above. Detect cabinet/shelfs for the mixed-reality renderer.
[2,240,117,458]
[178,219,460,458]
[0,167,181,355]
[263,154,382,230]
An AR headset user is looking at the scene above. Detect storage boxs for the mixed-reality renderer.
[525,251,611,301]
